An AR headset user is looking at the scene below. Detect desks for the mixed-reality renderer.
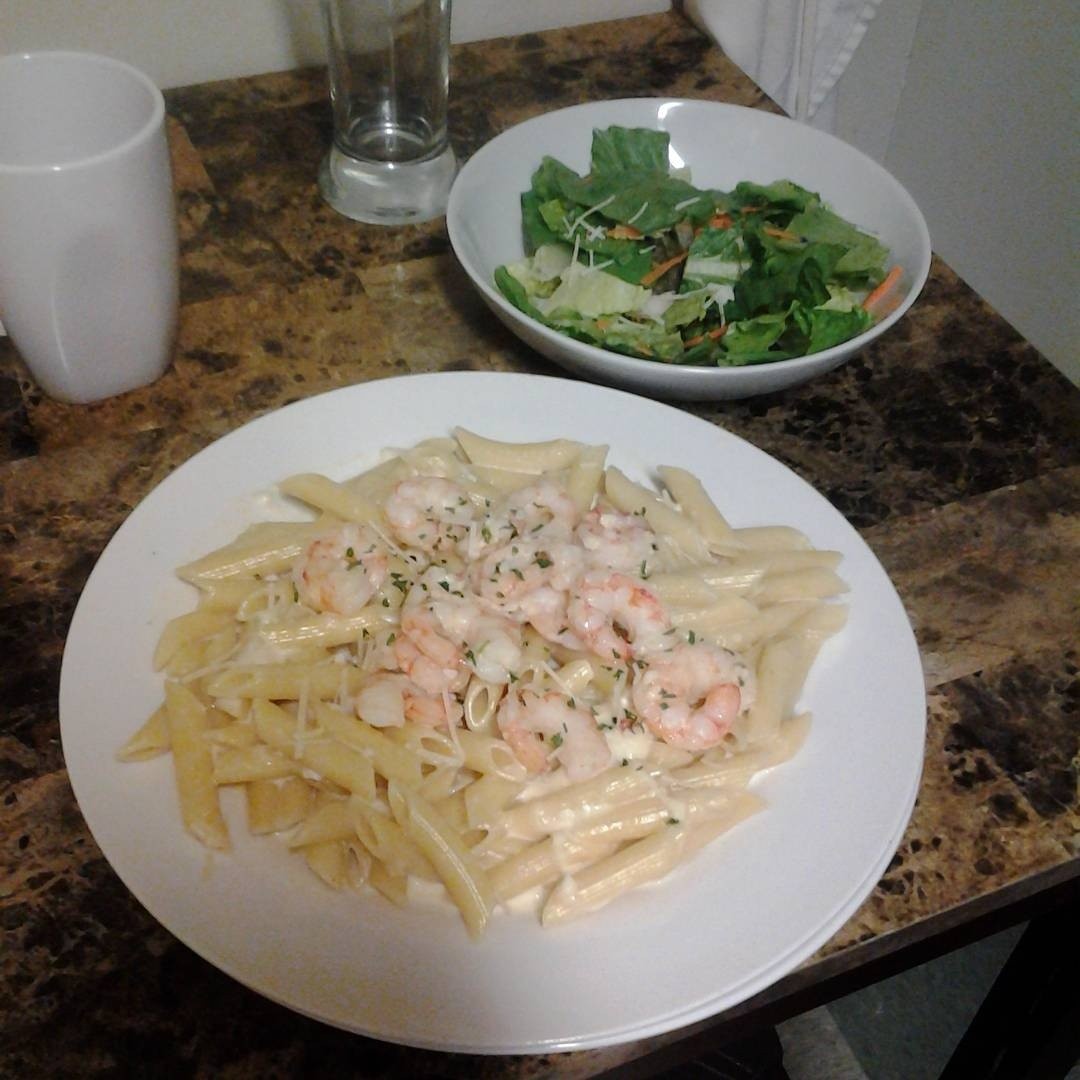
[0,10,1080,1080]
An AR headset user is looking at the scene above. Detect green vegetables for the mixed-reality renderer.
[495,125,884,368]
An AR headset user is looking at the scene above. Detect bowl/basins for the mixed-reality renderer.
[445,97,931,403]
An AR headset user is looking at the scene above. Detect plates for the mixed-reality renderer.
[57,369,928,1056]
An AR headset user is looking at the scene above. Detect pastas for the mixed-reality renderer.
[120,424,849,935]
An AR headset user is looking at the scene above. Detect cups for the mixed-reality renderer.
[0,51,177,404]
[315,0,464,227]
